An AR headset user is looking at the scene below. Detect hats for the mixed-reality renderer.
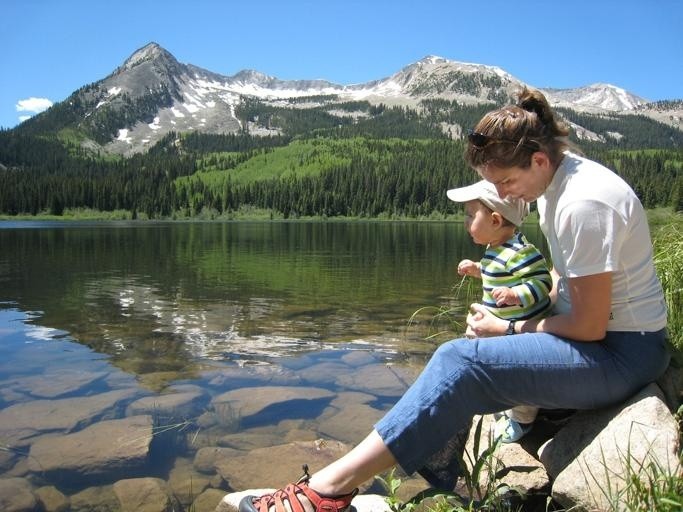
[446,179,530,227]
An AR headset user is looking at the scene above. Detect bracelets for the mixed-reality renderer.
[506,320,516,335]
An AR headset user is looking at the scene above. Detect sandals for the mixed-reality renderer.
[238,464,359,512]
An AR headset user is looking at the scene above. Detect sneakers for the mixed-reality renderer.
[500,419,533,444]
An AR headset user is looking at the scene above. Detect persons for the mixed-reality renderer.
[239,86,667,511]
[447,180,553,442]
